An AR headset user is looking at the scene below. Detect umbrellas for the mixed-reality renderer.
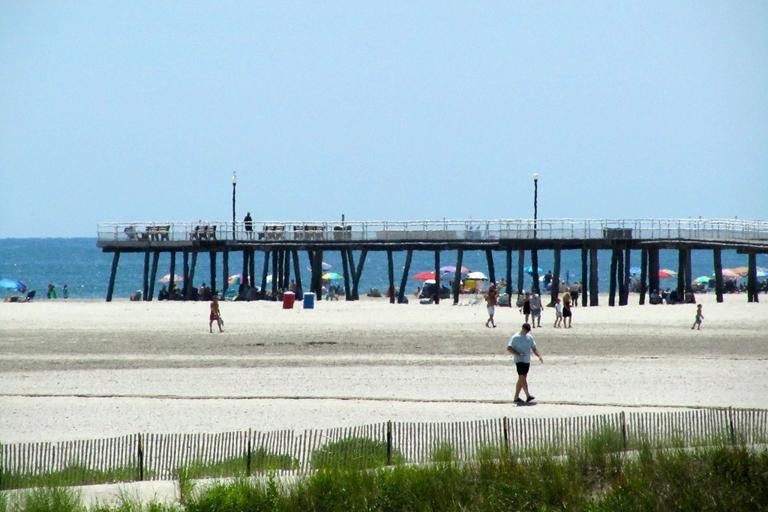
[307,262,332,271]
[267,274,285,284]
[647,269,678,278]
[321,273,344,280]
[630,267,642,275]
[694,275,714,284]
[160,272,184,283]
[414,266,488,281]
[228,274,250,285]
[712,266,768,276]
[0,277,27,293]
[523,265,544,276]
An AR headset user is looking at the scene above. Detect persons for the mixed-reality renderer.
[48,283,58,298]
[689,303,704,330]
[543,269,553,284]
[552,298,563,328]
[62,285,69,299]
[529,293,544,328]
[562,290,578,328]
[507,324,544,405]
[130,289,143,300]
[484,285,498,328]
[209,294,224,332]
[518,292,529,325]
[243,212,253,239]
[648,275,768,304]
[159,280,650,302]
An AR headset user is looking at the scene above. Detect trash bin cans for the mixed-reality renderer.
[282,291,296,309]
[303,292,314,308]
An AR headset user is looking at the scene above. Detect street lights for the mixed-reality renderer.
[231,175,238,240]
[532,173,539,239]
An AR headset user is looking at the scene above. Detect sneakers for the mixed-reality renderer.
[512,394,535,405]
